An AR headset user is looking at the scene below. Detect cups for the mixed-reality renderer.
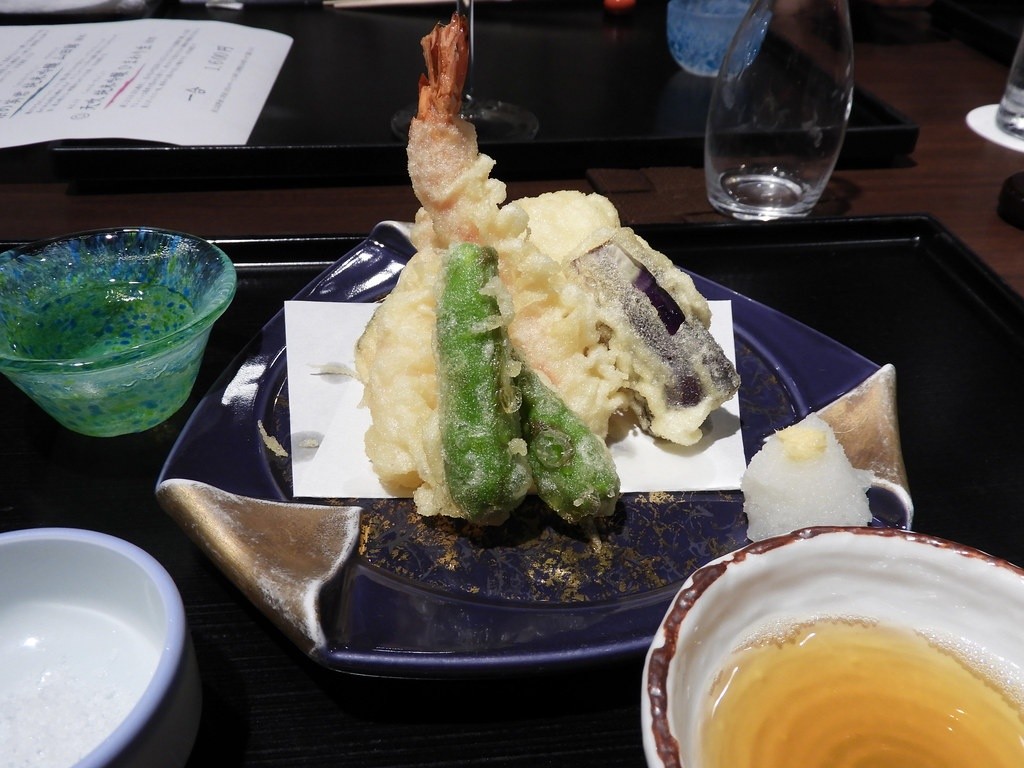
[998,34,1024,139]
[708,0,854,222]
[666,0,772,79]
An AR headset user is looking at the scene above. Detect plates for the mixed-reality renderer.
[155,220,914,674]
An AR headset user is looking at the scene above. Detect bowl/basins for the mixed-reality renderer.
[0,527,203,768]
[639,526,1024,768]
[0,225,236,439]
